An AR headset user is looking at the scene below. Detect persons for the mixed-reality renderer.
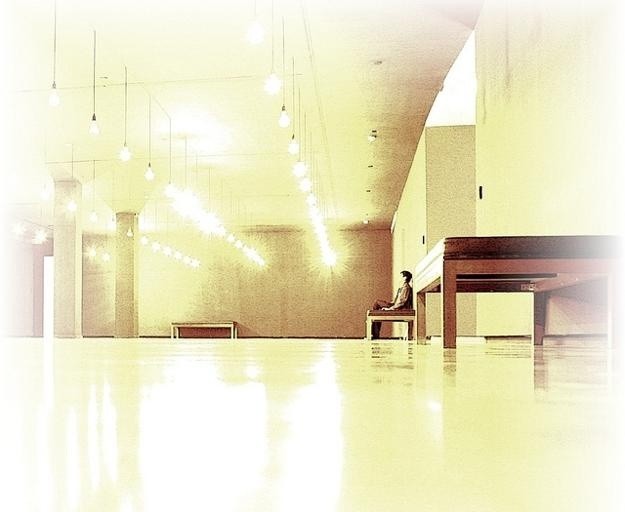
[371,271,412,338]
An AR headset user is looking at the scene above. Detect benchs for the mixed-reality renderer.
[366,310,414,341]
[413,235,617,349]
[169,322,237,338]
